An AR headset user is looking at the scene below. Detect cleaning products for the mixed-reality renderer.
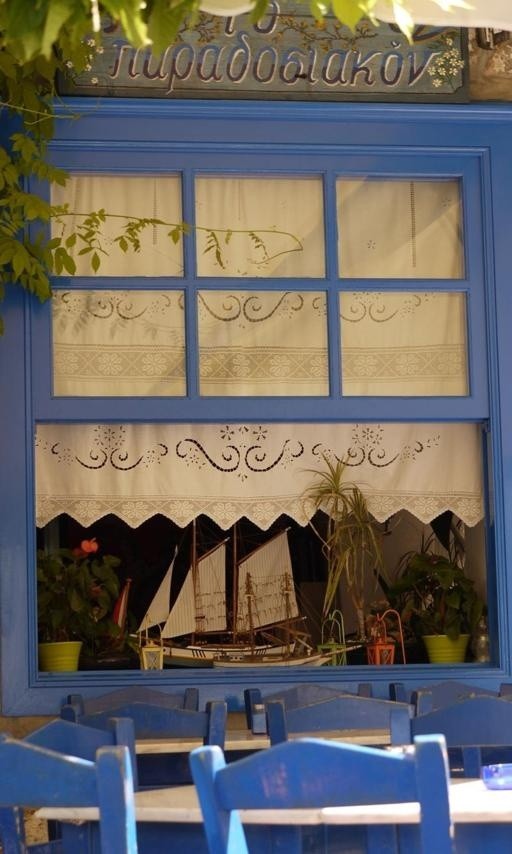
[470,616,490,663]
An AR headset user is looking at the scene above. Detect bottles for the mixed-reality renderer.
[473,613,490,663]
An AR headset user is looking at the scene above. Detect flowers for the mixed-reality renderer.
[38,531,116,639]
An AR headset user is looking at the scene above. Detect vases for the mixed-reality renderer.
[38,634,87,675]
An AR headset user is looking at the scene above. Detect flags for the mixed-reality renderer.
[114,585,129,628]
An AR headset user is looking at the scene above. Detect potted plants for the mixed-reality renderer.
[397,530,486,663]
